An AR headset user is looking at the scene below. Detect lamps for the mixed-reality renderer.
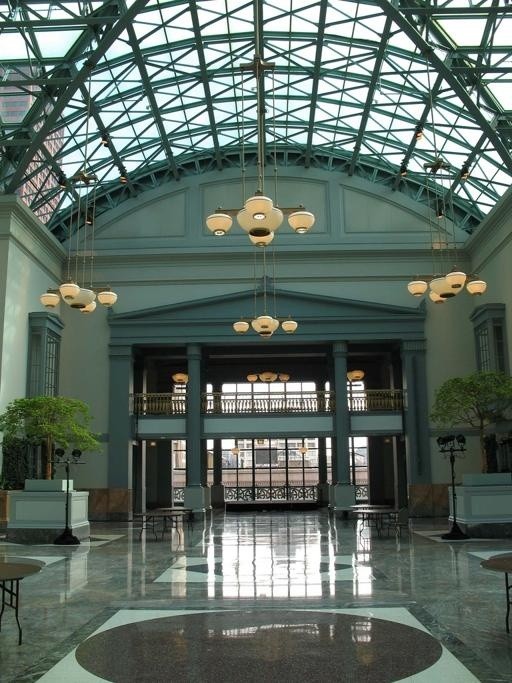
[39,57,119,314]
[203,52,316,337]
[404,47,486,305]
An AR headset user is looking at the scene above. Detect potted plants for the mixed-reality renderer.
[1,394,105,542]
[428,369,511,538]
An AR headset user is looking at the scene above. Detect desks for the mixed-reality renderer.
[350,503,398,537]
[0,562,42,646]
[134,507,195,543]
[479,557,512,634]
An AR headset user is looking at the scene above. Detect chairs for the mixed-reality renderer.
[387,506,410,537]
